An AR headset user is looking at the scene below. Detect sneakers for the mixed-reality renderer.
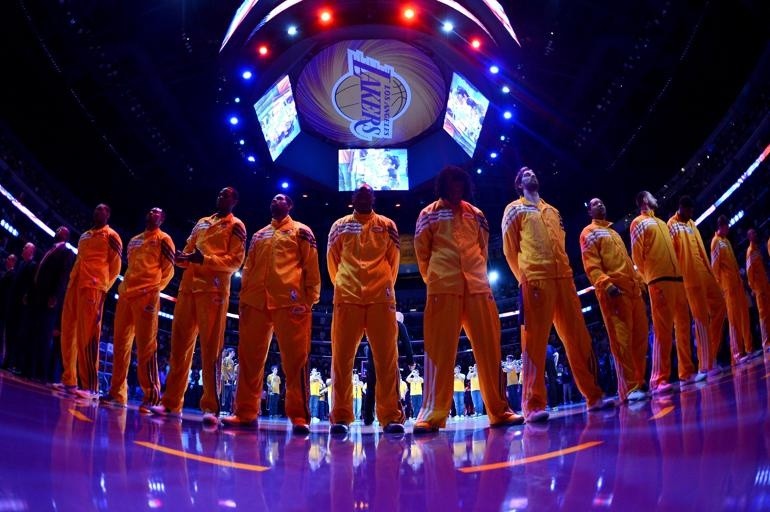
[149,405,184,418]
[736,355,749,365]
[75,389,100,401]
[490,414,524,428]
[138,402,151,413]
[526,410,550,423]
[330,422,349,435]
[626,390,647,402]
[413,422,440,436]
[679,373,707,388]
[586,399,616,413]
[748,349,762,360]
[292,423,311,436]
[52,383,78,394]
[384,422,406,434]
[221,416,259,430]
[202,412,218,425]
[650,383,673,396]
[102,399,128,409]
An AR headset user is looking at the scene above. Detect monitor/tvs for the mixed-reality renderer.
[254,74,301,162]
[338,149,409,191]
[442,71,489,158]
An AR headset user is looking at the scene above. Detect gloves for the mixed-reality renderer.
[608,286,621,298]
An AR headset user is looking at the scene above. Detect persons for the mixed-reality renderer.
[8,242,38,377]
[406,369,423,420]
[221,427,312,512]
[453,365,465,418]
[267,365,281,418]
[610,357,770,512]
[443,118,475,157]
[746,229,770,352]
[4,254,18,371]
[363,383,367,395]
[149,185,246,426]
[412,425,524,512]
[399,375,408,415]
[221,348,239,416]
[467,364,483,416]
[51,203,122,402]
[99,404,160,512]
[632,191,708,395]
[513,353,523,412]
[580,197,658,401]
[667,195,731,376]
[99,207,176,416]
[328,433,405,511]
[712,214,762,367]
[501,167,616,421]
[413,168,525,434]
[146,413,224,512]
[22,225,78,386]
[221,194,321,436]
[562,366,573,405]
[339,149,355,191]
[352,374,362,420]
[254,75,291,116]
[524,409,615,512]
[326,378,332,415]
[319,380,326,422]
[502,355,518,413]
[310,368,322,421]
[50,390,98,512]
[364,312,416,426]
[327,185,405,435]
